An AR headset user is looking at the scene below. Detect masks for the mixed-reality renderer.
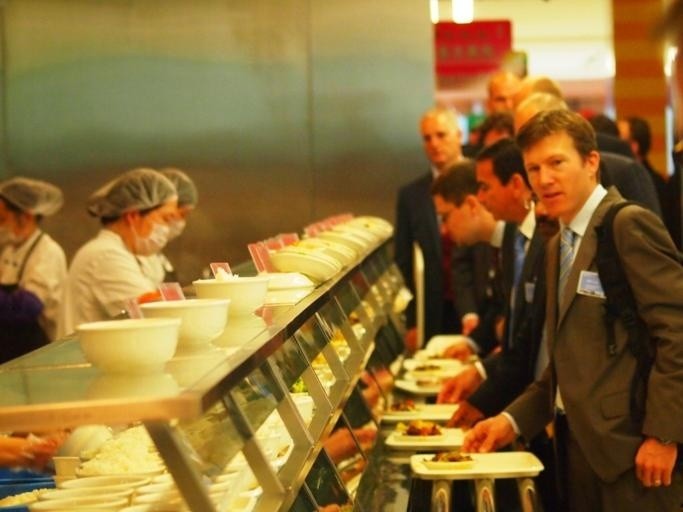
[0,214,36,246]
[127,212,171,257]
[172,220,186,241]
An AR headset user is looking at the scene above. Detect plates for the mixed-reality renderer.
[255,214,395,292]
[400,356,463,377]
[410,450,546,482]
[379,401,460,426]
[383,426,466,452]
[392,379,446,398]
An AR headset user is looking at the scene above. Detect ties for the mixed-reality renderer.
[509,234,525,290]
[557,228,574,301]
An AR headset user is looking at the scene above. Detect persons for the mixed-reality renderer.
[397,74,682,511]
[131,167,197,284]
[54,167,177,344]
[0,175,69,366]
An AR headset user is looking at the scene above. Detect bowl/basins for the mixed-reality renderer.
[74,317,183,379]
[140,297,230,349]
[27,446,168,512]
[193,274,269,318]
